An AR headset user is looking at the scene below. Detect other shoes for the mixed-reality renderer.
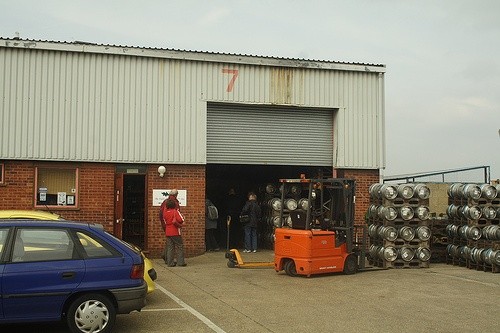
[243,249,251,253]
[252,249,256,252]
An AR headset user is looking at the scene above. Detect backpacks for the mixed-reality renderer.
[206,203,218,219]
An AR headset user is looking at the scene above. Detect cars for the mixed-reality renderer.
[0,209,157,296]
[0,218,149,333]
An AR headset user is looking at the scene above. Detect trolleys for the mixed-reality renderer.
[223,215,274,270]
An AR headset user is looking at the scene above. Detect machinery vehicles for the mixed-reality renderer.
[273,174,390,279]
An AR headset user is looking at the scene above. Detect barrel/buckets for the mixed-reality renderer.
[446,182,500,266]
[259,183,316,249]
[367,183,431,262]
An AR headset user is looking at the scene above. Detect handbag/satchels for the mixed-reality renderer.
[172,210,186,227]
[239,214,250,224]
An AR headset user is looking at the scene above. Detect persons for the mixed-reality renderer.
[160,188,180,265]
[241,192,262,252]
[205,193,221,251]
[163,199,187,266]
[225,187,244,249]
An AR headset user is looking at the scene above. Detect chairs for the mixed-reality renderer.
[11,239,25,262]
